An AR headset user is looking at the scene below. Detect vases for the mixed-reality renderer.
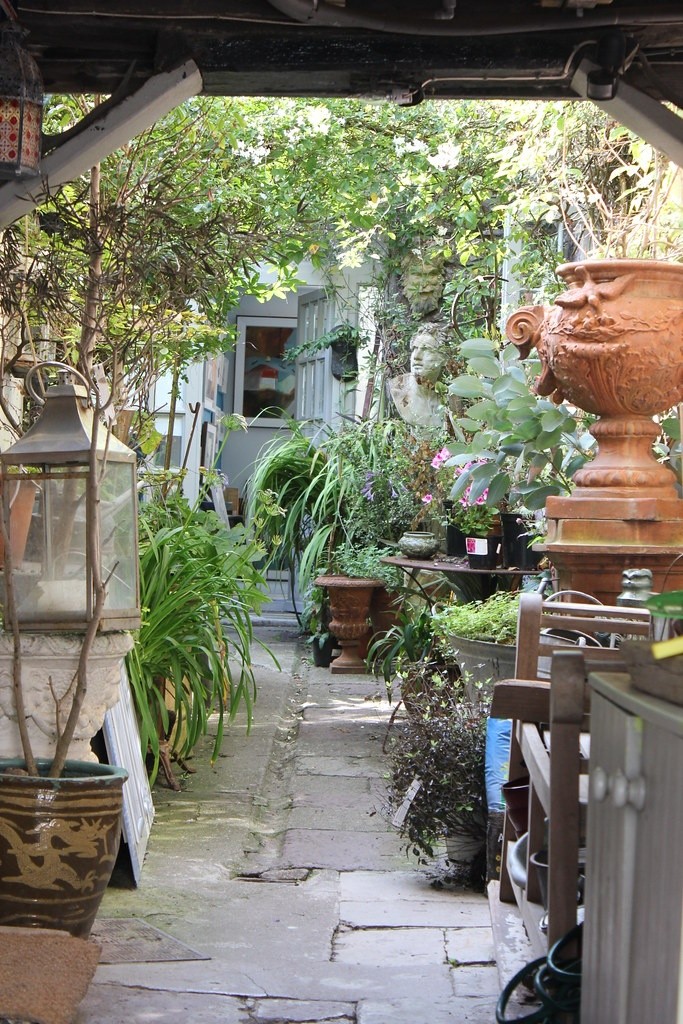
[466,534,501,570]
[498,512,547,569]
[399,531,439,558]
[201,501,234,512]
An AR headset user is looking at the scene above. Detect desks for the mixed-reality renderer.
[378,557,545,605]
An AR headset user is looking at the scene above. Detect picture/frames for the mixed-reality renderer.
[200,422,218,485]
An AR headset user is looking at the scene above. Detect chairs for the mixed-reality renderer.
[499,594,653,964]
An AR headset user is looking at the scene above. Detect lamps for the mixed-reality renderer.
[1,359,144,633]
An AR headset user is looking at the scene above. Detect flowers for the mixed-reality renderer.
[429,448,500,535]
[199,468,229,497]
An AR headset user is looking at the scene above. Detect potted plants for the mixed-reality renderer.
[1,123,321,935]
[431,590,523,702]
[370,664,489,861]
[302,541,408,673]
[368,608,464,739]
